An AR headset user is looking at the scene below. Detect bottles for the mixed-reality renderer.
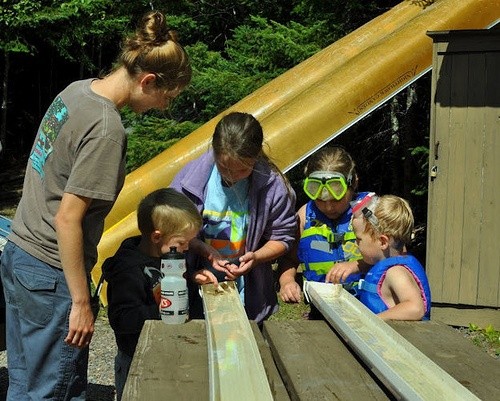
[160,246,189,323]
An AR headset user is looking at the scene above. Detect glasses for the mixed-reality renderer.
[303,170,353,201]
[350,192,384,234]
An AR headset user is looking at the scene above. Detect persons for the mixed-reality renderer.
[0,12,191,401]
[274,145,373,321]
[102,187,218,401]
[169,112,300,331]
[353,194,431,322]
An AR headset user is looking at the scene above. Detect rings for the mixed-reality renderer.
[223,263,232,267]
